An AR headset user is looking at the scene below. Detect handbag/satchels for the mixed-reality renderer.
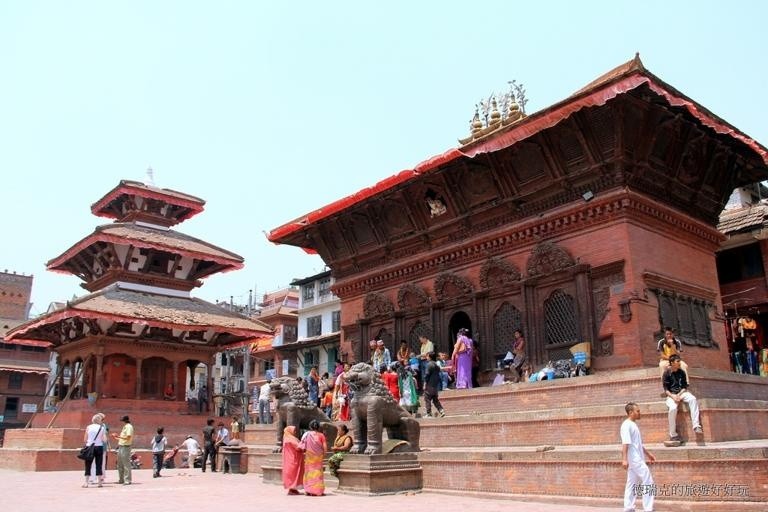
[454,343,467,353]
[77,447,94,460]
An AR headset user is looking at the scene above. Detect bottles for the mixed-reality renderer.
[496,360,501,369]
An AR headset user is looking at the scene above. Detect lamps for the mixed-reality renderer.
[581,189,594,203]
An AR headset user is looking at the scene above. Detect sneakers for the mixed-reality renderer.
[670,436,684,443]
[694,427,703,433]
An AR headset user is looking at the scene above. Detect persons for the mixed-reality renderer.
[281,426,304,496]
[111,415,134,485]
[257,327,480,426]
[88,413,112,485]
[162,382,211,415]
[201,418,240,473]
[617,401,659,512]
[656,326,690,398]
[81,415,106,488]
[662,354,704,445]
[301,419,329,496]
[508,329,529,383]
[328,424,355,479]
[176,434,204,469]
[150,426,168,479]
